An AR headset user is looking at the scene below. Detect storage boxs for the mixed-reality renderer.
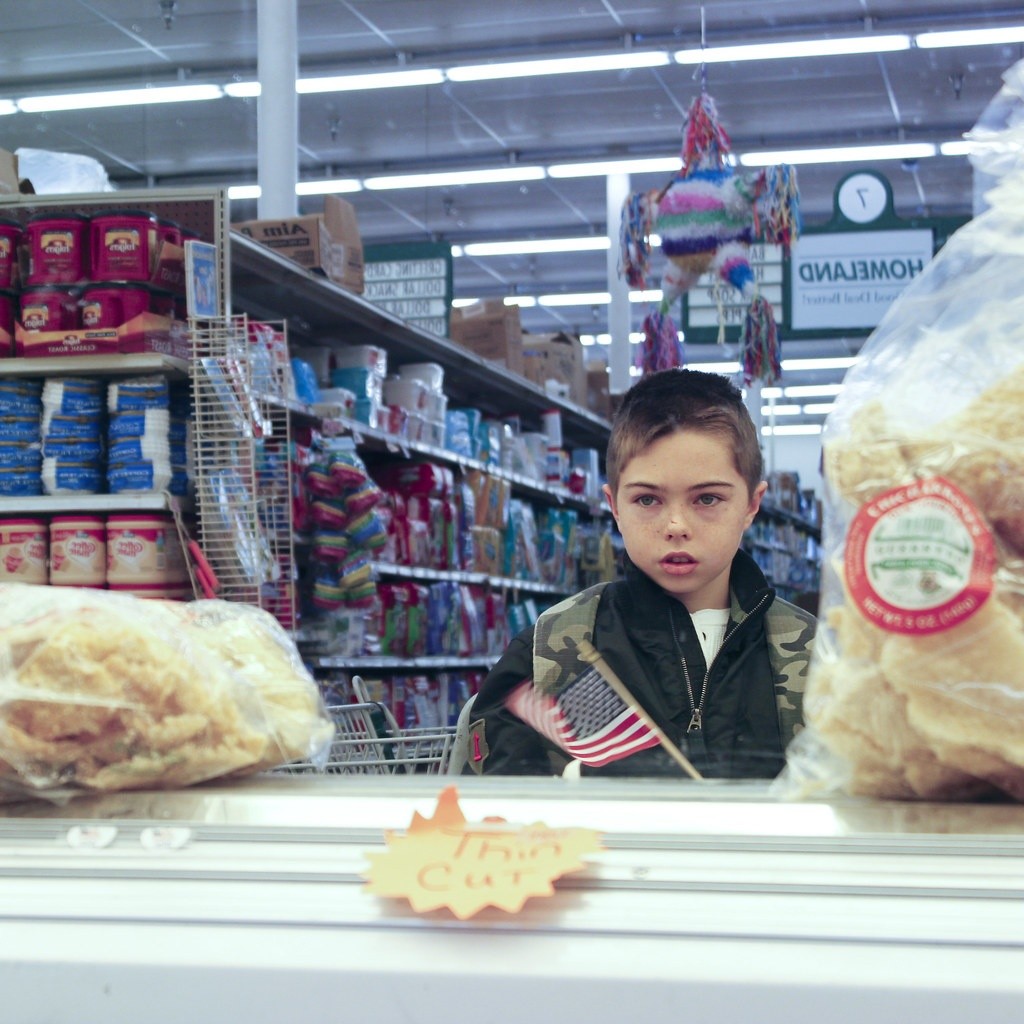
[448,296,614,425]
[229,194,365,295]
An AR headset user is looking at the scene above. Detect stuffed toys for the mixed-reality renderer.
[617,97,798,379]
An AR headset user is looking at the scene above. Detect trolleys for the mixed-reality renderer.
[264,675,457,775]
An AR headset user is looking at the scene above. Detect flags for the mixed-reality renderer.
[505,668,660,767]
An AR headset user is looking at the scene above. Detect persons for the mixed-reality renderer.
[467,368,820,779]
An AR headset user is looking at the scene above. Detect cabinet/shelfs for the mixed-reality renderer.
[1,184,822,773]
